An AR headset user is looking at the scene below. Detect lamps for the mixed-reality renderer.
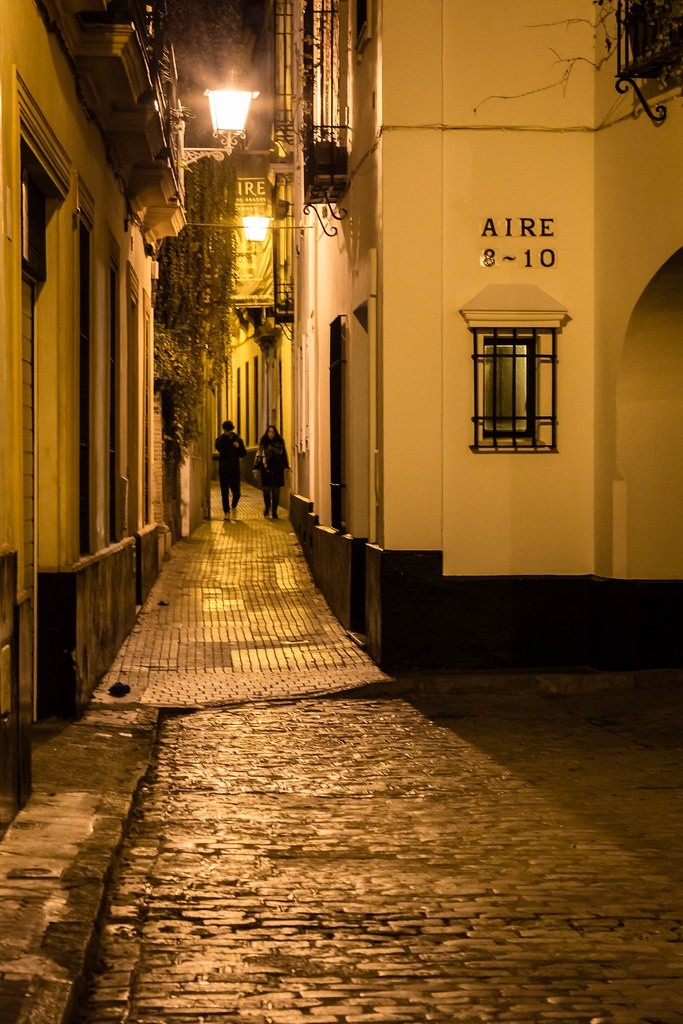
[274,199,295,220]
[241,210,275,242]
[182,67,261,166]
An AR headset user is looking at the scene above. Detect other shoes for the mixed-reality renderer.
[264,507,269,517]
[271,512,277,519]
[223,513,229,520]
[231,507,237,517]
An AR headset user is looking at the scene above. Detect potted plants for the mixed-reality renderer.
[314,128,338,165]
[594,0,683,95]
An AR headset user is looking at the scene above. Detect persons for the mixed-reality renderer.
[215,420,247,521]
[253,425,290,519]
